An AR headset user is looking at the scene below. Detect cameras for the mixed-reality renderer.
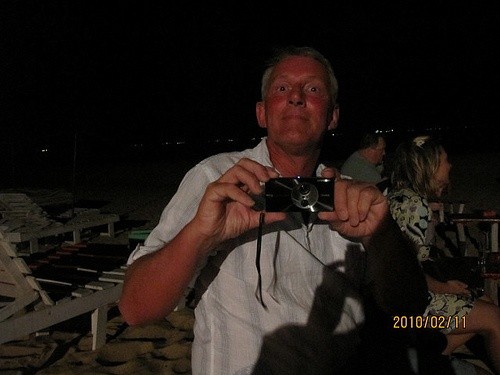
[265,176,335,212]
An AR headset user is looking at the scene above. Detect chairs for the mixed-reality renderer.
[0,194,131,350]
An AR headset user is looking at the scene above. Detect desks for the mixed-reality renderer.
[446,213,500,263]
